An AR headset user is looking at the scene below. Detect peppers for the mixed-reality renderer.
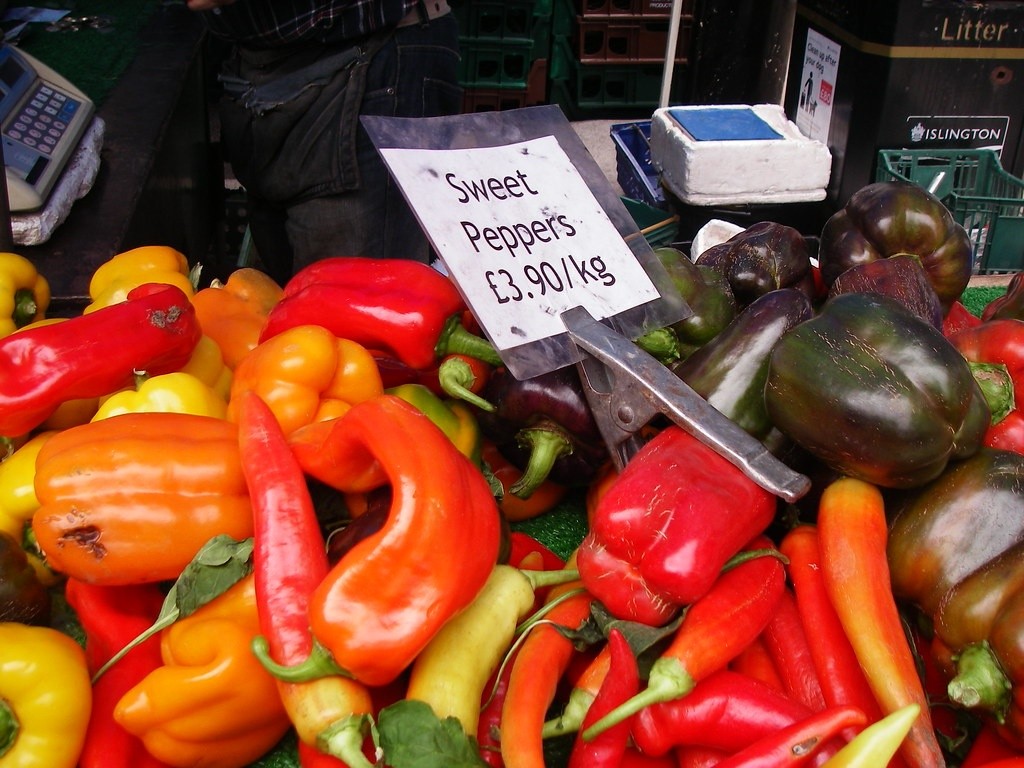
[0,177,1024,768]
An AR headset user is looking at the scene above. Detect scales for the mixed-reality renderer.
[0,43,96,213]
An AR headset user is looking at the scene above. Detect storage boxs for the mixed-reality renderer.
[649,100,833,203]
[448,0,697,123]
[874,147,1024,276]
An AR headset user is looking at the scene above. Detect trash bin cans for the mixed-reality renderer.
[785,0,1024,237]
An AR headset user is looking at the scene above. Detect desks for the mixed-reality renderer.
[9,2,209,309]
